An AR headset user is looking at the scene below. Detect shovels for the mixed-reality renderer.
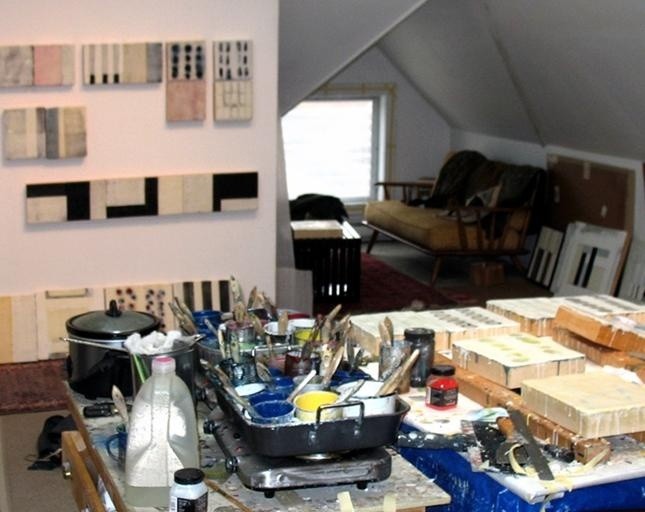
[494,416,529,466]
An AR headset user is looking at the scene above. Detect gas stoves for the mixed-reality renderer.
[192,362,391,491]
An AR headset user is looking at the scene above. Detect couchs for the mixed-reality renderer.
[362,153,536,284]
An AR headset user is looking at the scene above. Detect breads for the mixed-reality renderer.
[450,329,587,391]
[346,305,522,364]
[553,304,645,352]
[518,370,645,439]
[486,292,645,338]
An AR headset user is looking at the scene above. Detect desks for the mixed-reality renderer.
[61,379,452,512]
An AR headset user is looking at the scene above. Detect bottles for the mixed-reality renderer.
[426,364,459,411]
[169,468,208,512]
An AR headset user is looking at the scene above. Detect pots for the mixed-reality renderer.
[65,333,207,384]
[65,301,161,396]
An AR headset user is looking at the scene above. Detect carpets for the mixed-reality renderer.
[313,250,457,317]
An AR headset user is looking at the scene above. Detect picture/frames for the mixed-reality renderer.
[528,228,562,285]
[554,223,629,297]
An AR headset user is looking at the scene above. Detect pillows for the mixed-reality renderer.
[439,186,501,221]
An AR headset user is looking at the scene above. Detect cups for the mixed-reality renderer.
[193,309,411,423]
[105,423,128,471]
